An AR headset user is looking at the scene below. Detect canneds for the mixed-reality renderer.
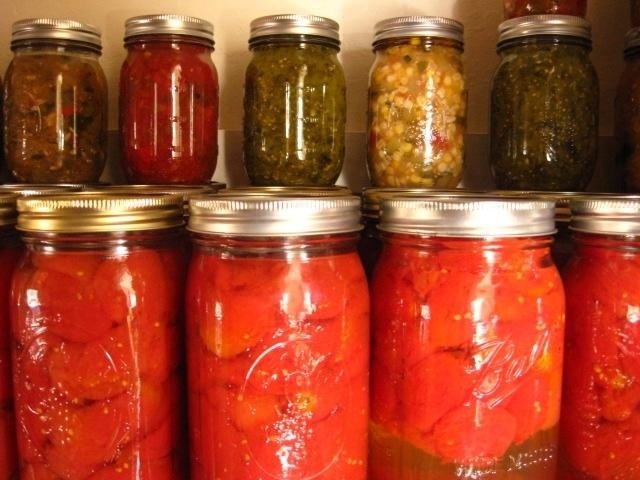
[361,189,567,479]
[182,187,372,480]
[1,17,108,184]
[242,13,347,187]
[542,193,640,480]
[366,14,469,189]
[489,13,600,192]
[118,13,220,186]
[1,185,215,478]
[617,26,640,195]
